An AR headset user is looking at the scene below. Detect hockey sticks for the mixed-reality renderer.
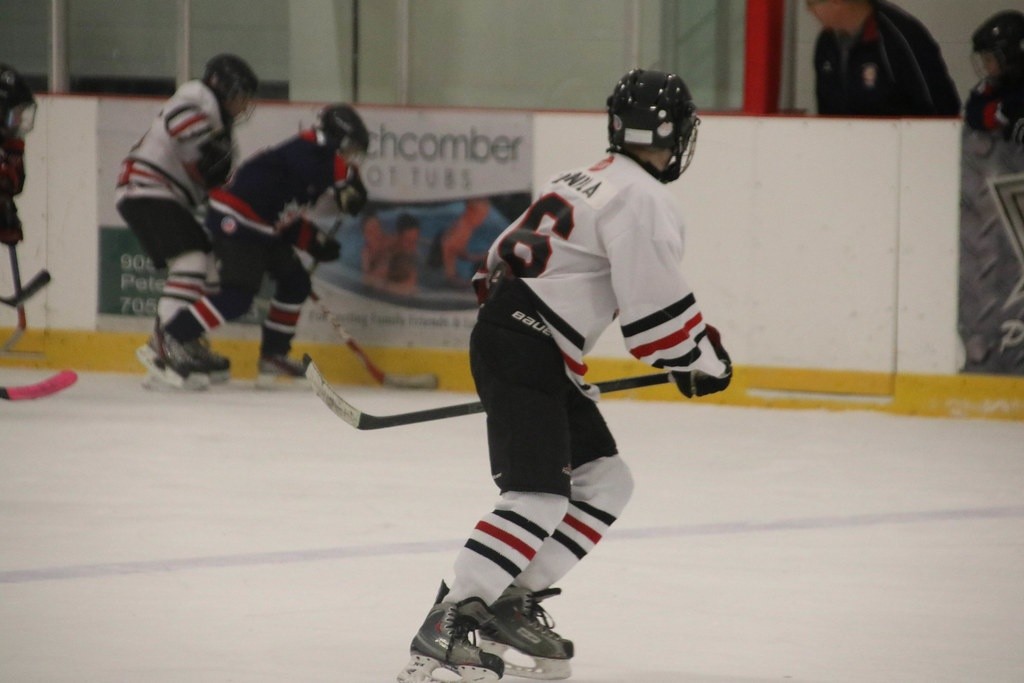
[0,243,77,399]
[282,235,440,388]
[300,350,677,430]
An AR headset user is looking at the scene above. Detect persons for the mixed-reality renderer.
[136,101,371,390]
[963,9,1024,140]
[113,52,260,385]
[358,198,491,289]
[0,63,38,245]
[396,67,733,683]
[805,0,962,119]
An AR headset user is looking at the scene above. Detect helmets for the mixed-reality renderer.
[606,68,702,183]
[0,64,38,140]
[311,103,368,167]
[201,53,259,128]
[968,10,1024,82]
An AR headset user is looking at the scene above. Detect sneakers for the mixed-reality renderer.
[476,582,575,679]
[253,352,317,391]
[394,580,505,683]
[134,327,232,392]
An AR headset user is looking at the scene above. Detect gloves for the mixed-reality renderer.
[0,210,23,247]
[334,164,367,216]
[306,221,340,264]
[668,322,734,398]
